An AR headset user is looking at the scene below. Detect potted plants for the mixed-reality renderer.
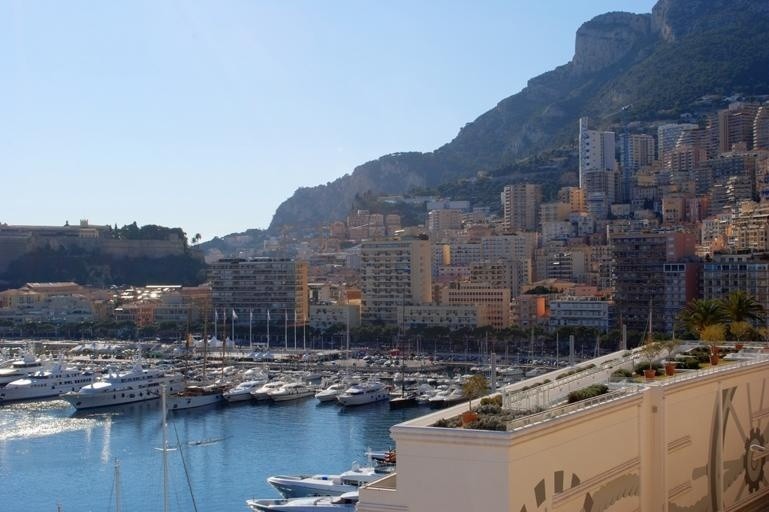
[643,320,750,381]
[461,373,489,428]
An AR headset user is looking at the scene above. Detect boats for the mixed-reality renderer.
[242,447,393,511]
